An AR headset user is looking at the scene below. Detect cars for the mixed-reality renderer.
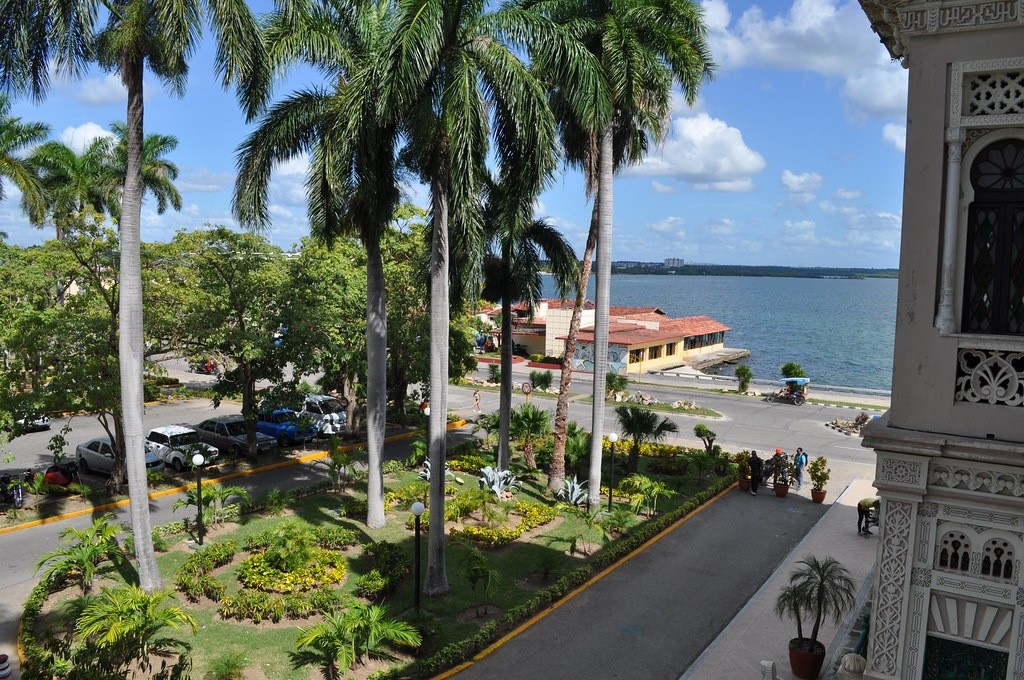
[144,425,219,472]
[192,415,279,458]
[256,408,321,449]
[76,436,166,483]
[4,411,52,432]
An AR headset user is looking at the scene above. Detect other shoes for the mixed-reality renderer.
[750,491,753,493]
[858,531,864,536]
[753,492,757,495]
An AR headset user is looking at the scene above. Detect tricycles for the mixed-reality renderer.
[767,377,811,406]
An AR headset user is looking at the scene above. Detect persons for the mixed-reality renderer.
[24,469,33,486]
[0,473,11,497]
[748,451,761,496]
[772,448,788,491]
[794,448,806,492]
[777,382,792,397]
[472,389,481,412]
[857,499,879,538]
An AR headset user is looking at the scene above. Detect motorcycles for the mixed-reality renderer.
[189,353,220,375]
[22,453,84,495]
[0,471,31,508]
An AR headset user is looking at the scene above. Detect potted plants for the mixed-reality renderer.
[769,457,801,497]
[773,552,859,678]
[733,449,753,491]
[806,456,831,503]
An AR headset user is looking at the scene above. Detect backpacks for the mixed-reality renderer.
[796,453,808,466]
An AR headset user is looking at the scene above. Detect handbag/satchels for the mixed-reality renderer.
[750,472,763,483]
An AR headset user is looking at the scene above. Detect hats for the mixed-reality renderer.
[776,448,782,454]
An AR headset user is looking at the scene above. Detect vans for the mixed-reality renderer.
[278,395,349,439]
